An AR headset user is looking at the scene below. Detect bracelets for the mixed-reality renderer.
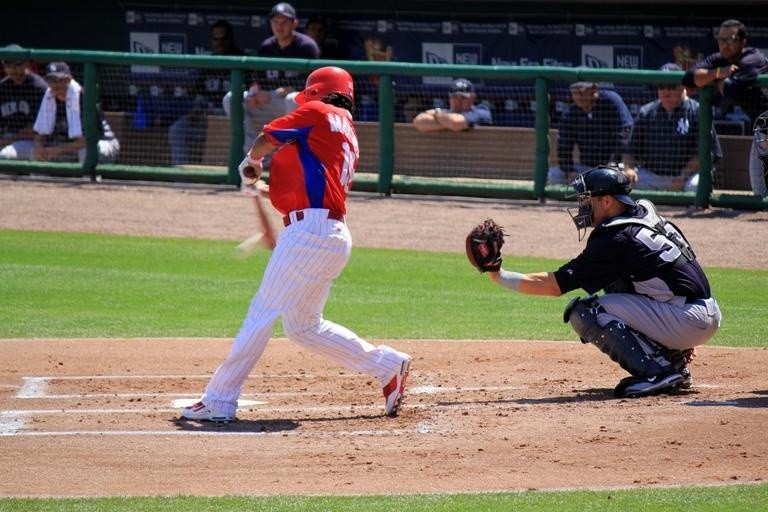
[497,271,522,291]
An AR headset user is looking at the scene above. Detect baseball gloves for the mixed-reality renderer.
[466,220,503,272]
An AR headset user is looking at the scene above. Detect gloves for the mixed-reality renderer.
[237,148,264,185]
[239,179,266,197]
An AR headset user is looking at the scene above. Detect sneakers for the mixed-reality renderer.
[383,354,412,416]
[612,370,684,398]
[673,359,693,390]
[182,399,236,422]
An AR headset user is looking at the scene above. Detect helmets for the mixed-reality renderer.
[564,164,640,242]
[752,110,767,176]
[294,66,355,107]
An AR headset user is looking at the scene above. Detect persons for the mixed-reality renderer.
[412,78,493,132]
[403,93,425,123]
[546,19,768,196]
[365,37,394,87]
[0,44,121,164]
[167,0,357,164]
[179,66,411,425]
[465,165,722,398]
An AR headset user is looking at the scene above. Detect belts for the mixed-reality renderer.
[684,297,705,306]
[282,210,346,227]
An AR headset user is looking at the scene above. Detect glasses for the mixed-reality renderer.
[716,34,742,44]
[47,77,63,83]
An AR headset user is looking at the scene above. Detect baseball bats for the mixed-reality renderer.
[243,167,276,251]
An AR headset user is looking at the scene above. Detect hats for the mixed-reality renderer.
[447,77,474,98]
[568,65,594,89]
[268,2,297,20]
[43,62,70,80]
[657,62,683,70]
